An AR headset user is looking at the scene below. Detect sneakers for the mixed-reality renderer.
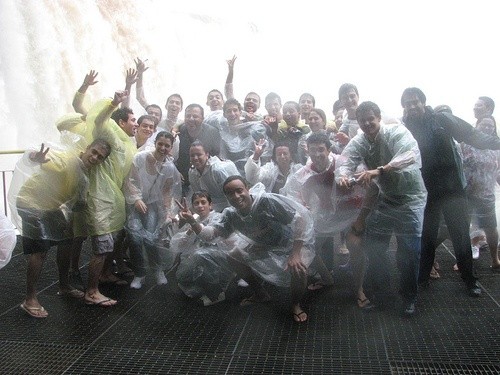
[130,275,145,290]
[154,271,168,285]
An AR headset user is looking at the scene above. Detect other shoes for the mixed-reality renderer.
[112,255,137,280]
[470,284,481,296]
[69,270,81,284]
[307,272,334,291]
[401,304,414,317]
[336,248,351,268]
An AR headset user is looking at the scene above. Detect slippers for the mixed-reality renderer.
[357,298,370,309]
[57,289,84,298]
[100,278,129,287]
[429,271,439,280]
[239,297,272,307]
[20,304,48,317]
[85,297,118,306]
[292,311,307,324]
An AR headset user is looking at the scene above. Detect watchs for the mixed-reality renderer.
[377,166,384,174]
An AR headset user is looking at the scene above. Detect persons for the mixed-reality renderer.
[16,141,110,318]
[398,88,500,296]
[122,131,185,289]
[334,101,427,314]
[175,175,308,323]
[453,115,500,270]
[83,89,138,306]
[244,138,306,194]
[55,54,453,289]
[177,190,227,305]
[286,133,372,308]
[474,96,497,137]
